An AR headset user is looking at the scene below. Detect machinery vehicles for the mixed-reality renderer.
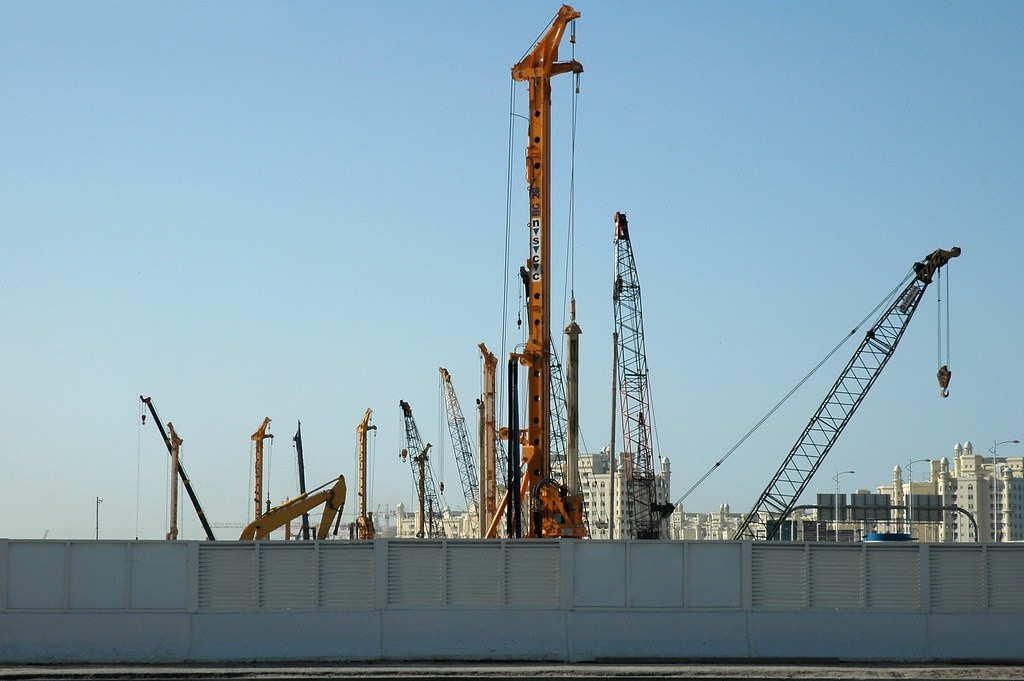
[501,4,585,539]
[240,474,346,541]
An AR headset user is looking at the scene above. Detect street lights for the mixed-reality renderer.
[988,440,1020,542]
[833,471,855,542]
[905,458,931,538]
